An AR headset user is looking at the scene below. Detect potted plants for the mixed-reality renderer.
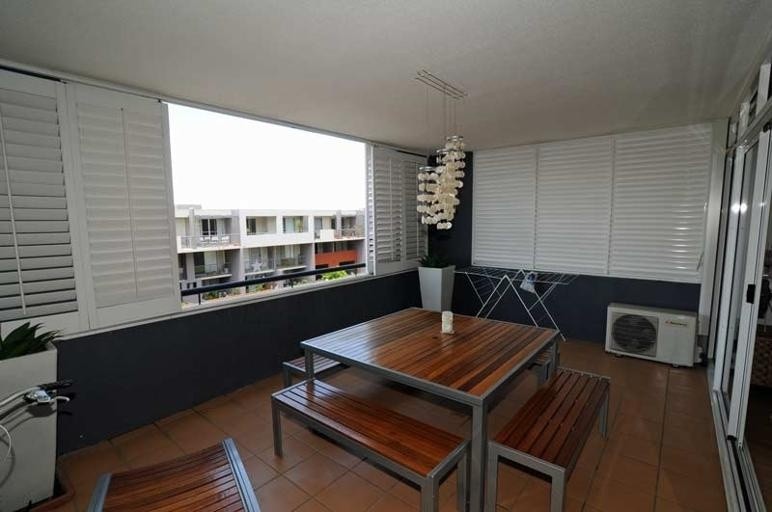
[0,320,75,512]
[416,254,456,312]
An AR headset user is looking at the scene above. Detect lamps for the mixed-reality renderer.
[414,68,469,228]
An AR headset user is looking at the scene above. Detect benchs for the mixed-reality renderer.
[484,368,610,510]
[90,440,259,512]
[283,352,352,384]
[271,377,472,512]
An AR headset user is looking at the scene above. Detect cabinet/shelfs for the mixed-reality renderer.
[453,265,580,343]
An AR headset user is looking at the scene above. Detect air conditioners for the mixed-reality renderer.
[604,302,703,368]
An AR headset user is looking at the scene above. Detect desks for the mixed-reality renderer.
[300,307,561,510]
